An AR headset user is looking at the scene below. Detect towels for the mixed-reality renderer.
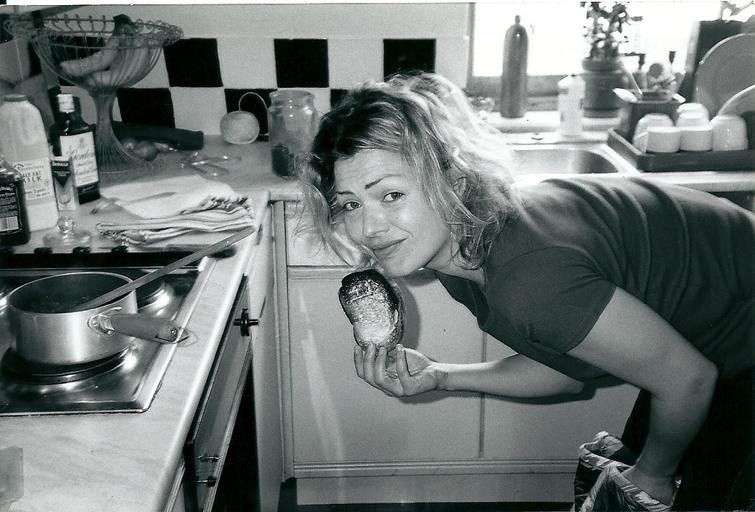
[101,175,245,221]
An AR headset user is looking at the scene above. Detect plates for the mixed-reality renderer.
[716,85,755,149]
[695,32,755,115]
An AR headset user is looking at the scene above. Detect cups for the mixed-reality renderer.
[710,114,748,152]
[633,104,713,152]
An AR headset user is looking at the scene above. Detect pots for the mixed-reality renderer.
[6,272,190,367]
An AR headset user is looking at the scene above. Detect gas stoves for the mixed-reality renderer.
[0,255,208,412]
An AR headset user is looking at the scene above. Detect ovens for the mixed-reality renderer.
[181,277,259,510]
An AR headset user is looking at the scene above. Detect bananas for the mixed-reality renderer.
[59,12,151,88]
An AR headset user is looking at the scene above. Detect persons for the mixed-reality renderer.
[290,69,753,511]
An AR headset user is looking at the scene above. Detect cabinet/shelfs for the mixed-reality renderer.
[246,209,282,511]
[280,199,640,482]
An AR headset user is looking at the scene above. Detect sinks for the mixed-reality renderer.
[452,136,621,181]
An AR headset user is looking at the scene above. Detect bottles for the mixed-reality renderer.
[0,157,30,246]
[267,89,319,180]
[49,93,100,205]
[41,217,95,253]
[557,72,588,138]
[498,16,529,118]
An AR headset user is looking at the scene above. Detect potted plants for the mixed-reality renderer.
[576,1,642,117]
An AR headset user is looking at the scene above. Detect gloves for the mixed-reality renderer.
[560,429,681,511]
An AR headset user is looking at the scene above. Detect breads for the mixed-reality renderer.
[338,268,404,353]
